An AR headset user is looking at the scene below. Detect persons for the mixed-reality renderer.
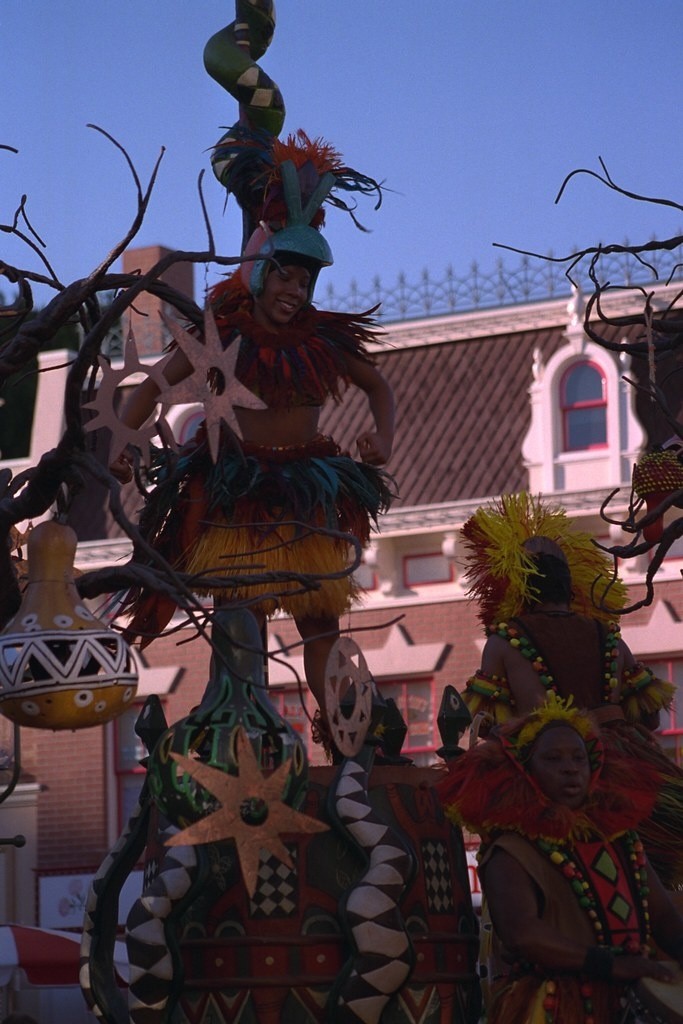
[473,535,661,733]
[479,697,683,1024]
[107,218,397,769]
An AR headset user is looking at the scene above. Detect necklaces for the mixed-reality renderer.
[536,830,652,960]
[484,619,620,704]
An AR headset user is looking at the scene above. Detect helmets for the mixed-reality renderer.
[240,129,385,314]
